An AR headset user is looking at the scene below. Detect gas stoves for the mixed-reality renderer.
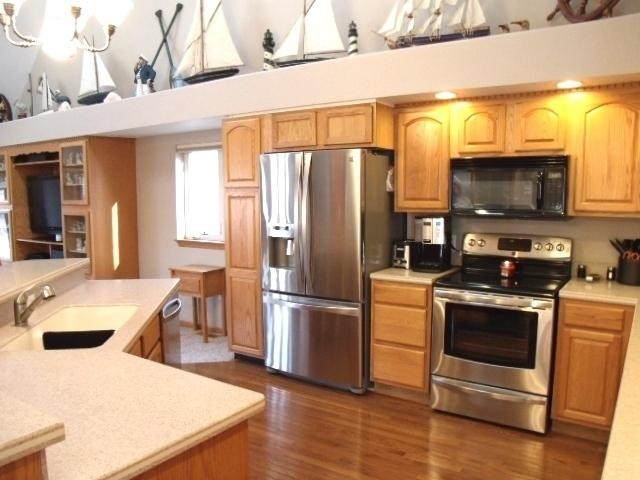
[435,267,569,296]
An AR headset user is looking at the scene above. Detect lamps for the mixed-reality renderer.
[0,2,119,59]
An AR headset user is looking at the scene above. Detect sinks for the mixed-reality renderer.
[0,303,140,354]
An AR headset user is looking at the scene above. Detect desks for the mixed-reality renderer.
[169,266,226,343]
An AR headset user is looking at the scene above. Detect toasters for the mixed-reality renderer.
[392,239,414,268]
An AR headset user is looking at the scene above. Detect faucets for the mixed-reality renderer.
[13,280,56,326]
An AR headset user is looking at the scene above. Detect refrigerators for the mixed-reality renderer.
[258,148,405,394]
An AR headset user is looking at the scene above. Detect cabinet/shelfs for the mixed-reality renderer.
[63,138,140,280]
[567,83,640,218]
[0,149,17,259]
[224,118,262,358]
[549,296,633,444]
[453,91,572,156]
[392,103,450,212]
[128,313,165,361]
[268,102,393,151]
[369,261,461,407]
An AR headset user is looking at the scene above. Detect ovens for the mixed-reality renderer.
[449,155,569,220]
[431,286,556,435]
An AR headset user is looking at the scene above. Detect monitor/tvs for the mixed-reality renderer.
[26,176,62,234]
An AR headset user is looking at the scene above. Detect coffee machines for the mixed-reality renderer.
[412,216,447,271]
